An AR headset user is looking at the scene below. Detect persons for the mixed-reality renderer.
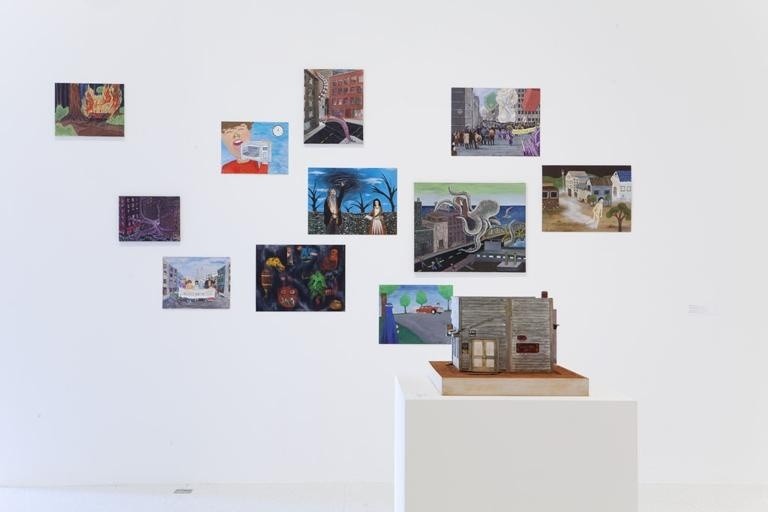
[324,182,344,233]
[367,198,386,234]
[452,126,494,149]
